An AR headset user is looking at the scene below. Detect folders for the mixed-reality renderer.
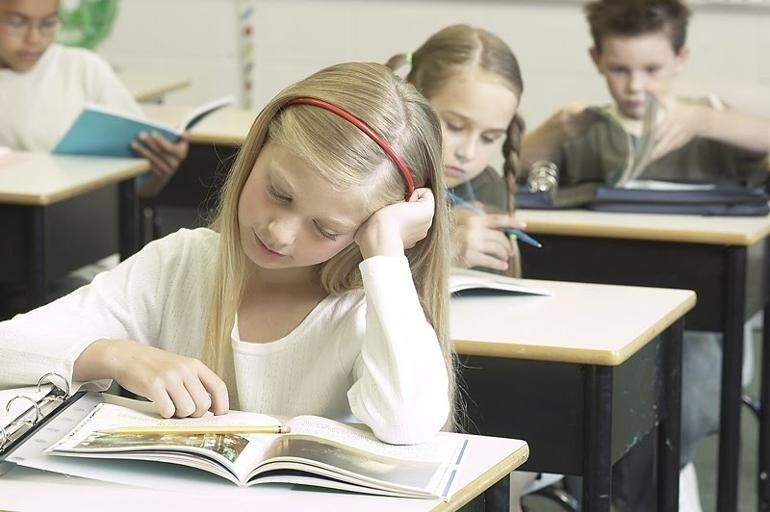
[516,182,769,215]
[1,383,178,484]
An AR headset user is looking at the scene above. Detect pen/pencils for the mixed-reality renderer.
[91,425,293,436]
[446,191,544,249]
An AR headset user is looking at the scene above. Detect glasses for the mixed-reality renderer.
[0,17,64,38]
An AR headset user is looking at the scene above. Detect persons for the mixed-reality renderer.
[0,62,481,446]
[0,0,190,202]
[516,0,766,509]
[385,24,526,280]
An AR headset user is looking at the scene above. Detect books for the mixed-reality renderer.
[516,87,770,217]
[447,267,551,297]
[44,393,469,503]
[51,97,240,192]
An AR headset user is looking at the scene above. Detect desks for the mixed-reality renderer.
[114,75,191,106]
[139,105,263,208]
[0,151,151,308]
[449,277,697,512]
[516,209,770,512]
[0,392,530,512]
